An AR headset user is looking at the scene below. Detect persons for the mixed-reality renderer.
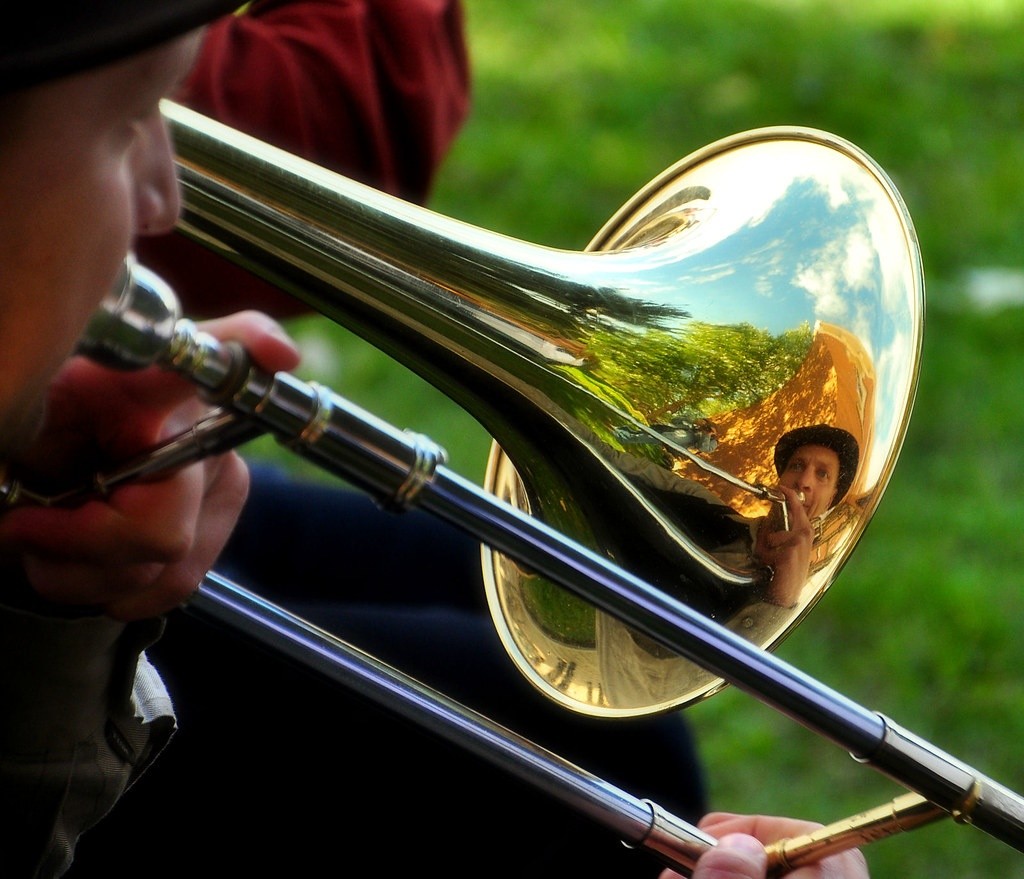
[0,0,875,878]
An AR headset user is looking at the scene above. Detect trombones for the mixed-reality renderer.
[1,92,1024,879]
[584,384,812,537]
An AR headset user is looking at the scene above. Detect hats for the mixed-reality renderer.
[774,423,859,510]
[0,0,249,87]
